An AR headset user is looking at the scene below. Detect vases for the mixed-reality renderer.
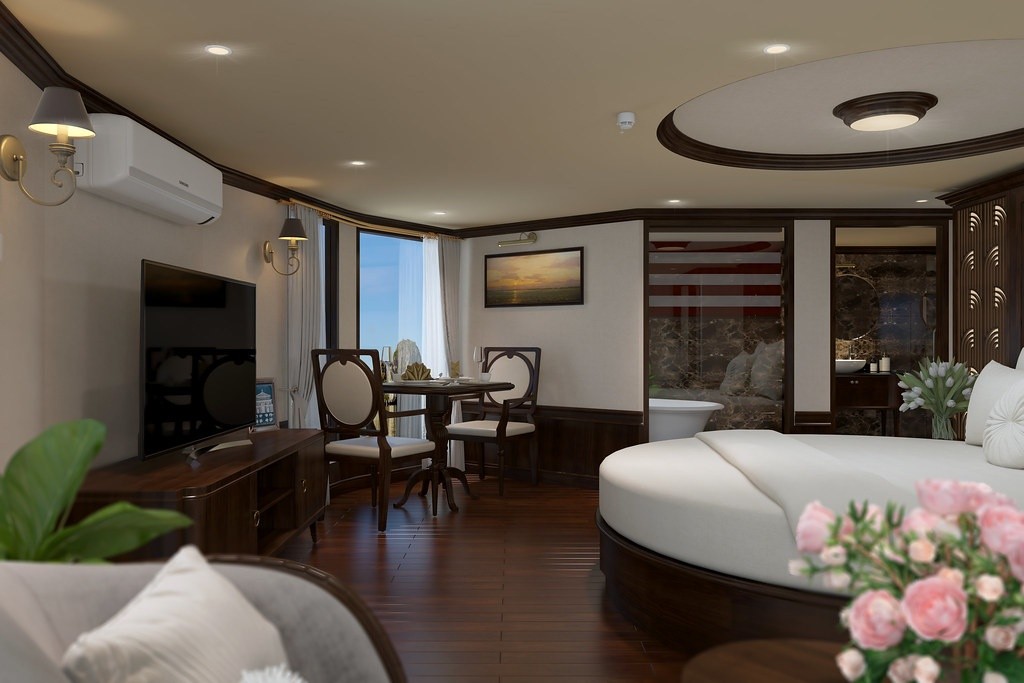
[930,411,959,442]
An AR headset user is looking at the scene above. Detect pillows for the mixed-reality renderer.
[1011,352,1024,382]
[976,382,1024,470]
[63,540,316,678]
[964,360,1019,444]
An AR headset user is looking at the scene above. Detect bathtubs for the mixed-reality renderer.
[648,398,725,443]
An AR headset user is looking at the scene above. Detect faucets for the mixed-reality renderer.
[848,345,858,360]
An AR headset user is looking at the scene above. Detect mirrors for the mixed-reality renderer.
[834,268,883,359]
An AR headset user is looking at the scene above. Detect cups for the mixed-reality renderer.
[879,360,881,371]
[870,363,877,371]
[481,373,490,381]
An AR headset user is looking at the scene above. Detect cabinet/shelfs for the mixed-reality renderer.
[65,425,331,560]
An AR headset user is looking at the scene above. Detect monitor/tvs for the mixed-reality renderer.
[136,259,256,463]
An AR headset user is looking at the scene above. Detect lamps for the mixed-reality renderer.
[263,218,308,275]
[1,86,99,204]
[496,228,538,248]
[833,92,938,136]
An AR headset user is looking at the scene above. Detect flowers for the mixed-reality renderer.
[784,478,1024,682]
[894,362,976,418]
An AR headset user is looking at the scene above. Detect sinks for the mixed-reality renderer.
[835,360,866,373]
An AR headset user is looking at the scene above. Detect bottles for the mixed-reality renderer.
[881,349,890,371]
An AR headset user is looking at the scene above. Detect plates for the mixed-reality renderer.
[399,380,445,386]
[444,378,473,382]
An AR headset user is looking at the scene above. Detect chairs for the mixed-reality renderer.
[442,342,551,495]
[304,346,439,533]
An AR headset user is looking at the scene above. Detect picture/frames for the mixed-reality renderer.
[483,246,586,309]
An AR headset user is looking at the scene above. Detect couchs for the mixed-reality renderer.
[1,555,423,682]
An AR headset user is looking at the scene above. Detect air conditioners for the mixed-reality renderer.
[71,114,223,228]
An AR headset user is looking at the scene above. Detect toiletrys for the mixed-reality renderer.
[879,352,890,372]
[870,358,877,372]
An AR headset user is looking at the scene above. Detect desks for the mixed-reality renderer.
[832,371,899,434]
[378,377,518,515]
[678,633,881,683]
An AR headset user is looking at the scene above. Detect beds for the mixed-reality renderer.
[599,350,1024,645]
